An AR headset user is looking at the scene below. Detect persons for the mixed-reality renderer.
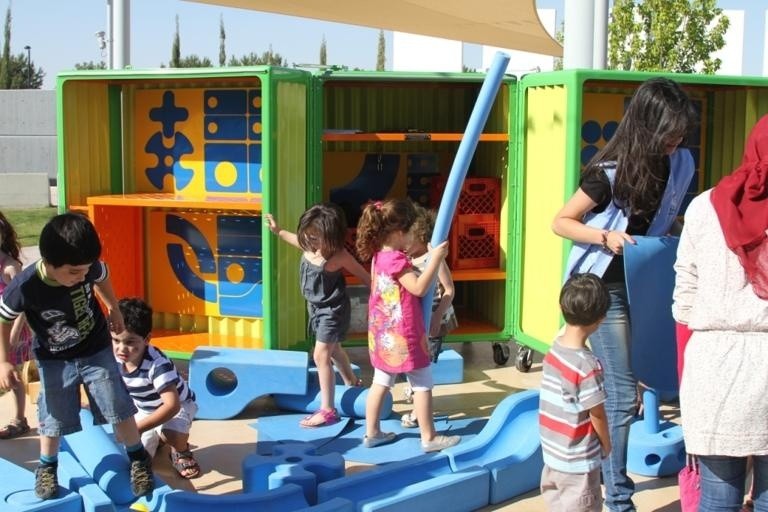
[355,195,461,454]
[539,273,613,512]
[401,204,454,428]
[551,76,700,511]
[1,213,156,499]
[261,204,371,428]
[673,114,768,512]
[105,297,200,479]
[1,213,36,439]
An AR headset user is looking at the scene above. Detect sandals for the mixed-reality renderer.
[0,416,31,440]
[299,407,340,428]
[169,448,201,480]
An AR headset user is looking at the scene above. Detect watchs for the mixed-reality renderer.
[599,231,610,254]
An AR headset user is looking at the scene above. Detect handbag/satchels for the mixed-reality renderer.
[676,453,703,512]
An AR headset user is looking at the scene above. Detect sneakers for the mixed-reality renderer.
[33,463,59,498]
[422,433,461,453]
[362,431,396,448]
[128,461,154,497]
